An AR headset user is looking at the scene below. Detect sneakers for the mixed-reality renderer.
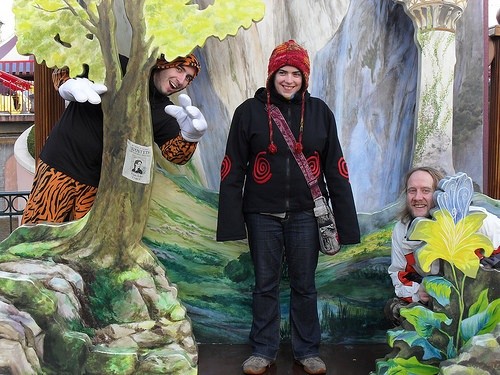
[302,357,327,374]
[242,357,271,375]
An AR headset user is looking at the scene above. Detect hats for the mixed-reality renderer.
[266,40,311,154]
[154,53,200,96]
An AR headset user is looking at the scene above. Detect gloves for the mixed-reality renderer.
[164,94,207,143]
[58,78,107,106]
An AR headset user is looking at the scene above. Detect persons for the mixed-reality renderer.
[216,39,362,374]
[385,167,500,327]
[132,158,144,175]
[22,49,206,225]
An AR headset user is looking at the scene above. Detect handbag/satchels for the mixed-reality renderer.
[312,194,342,255]
